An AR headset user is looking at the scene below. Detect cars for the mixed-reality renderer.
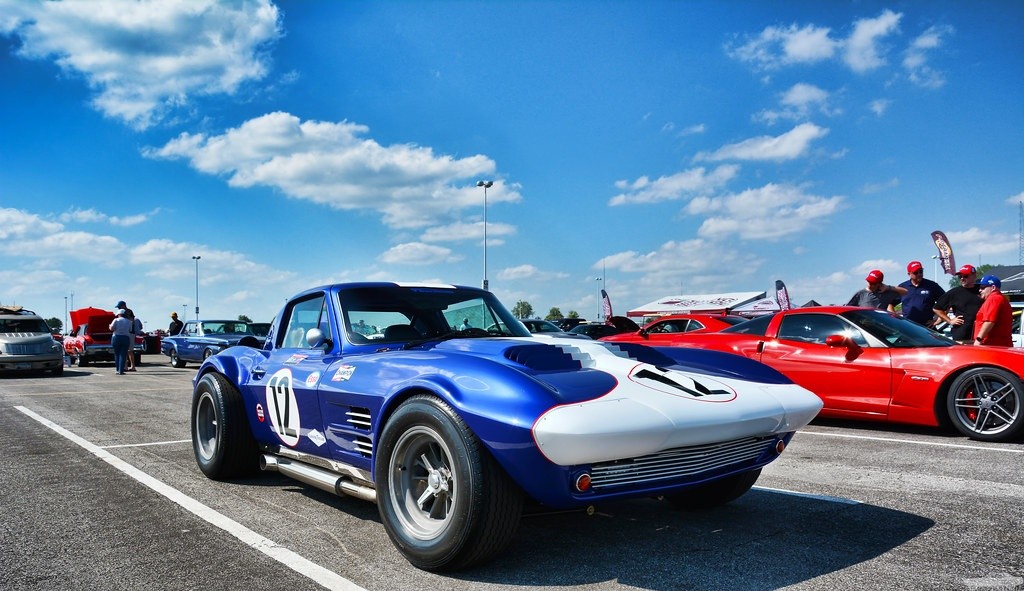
[50,327,63,341]
[291,320,387,341]
[483,316,641,341]
[138,329,169,354]
[594,311,752,342]
[215,322,272,337]
[887,299,1024,349]
[61,307,148,368]
[158,319,267,369]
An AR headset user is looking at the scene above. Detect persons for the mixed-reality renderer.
[887,261,950,342]
[525,324,533,332]
[65,326,81,364]
[169,312,183,336]
[115,301,137,371]
[846,270,908,310]
[224,323,233,332]
[109,308,133,375]
[459,317,472,331]
[451,325,457,330]
[661,322,680,332]
[134,317,145,366]
[971,274,1014,347]
[933,265,986,344]
[355,319,368,335]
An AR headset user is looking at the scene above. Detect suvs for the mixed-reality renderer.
[0,307,64,377]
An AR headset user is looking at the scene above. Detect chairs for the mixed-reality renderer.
[384,324,423,340]
[195,329,208,333]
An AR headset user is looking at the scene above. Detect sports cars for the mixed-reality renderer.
[669,302,1024,443]
[189,280,825,573]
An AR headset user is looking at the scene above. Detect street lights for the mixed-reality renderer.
[594,277,602,321]
[183,304,188,323]
[64,296,67,334]
[930,255,941,282]
[191,255,202,320]
[475,180,495,330]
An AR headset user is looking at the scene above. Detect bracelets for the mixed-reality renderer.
[886,284,891,292]
[976,337,982,342]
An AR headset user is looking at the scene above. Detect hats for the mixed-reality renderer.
[115,309,126,316]
[974,275,1001,288]
[956,265,976,276]
[865,270,883,284]
[115,301,126,308]
[907,261,923,273]
[170,312,177,316]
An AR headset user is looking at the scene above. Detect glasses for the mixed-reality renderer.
[913,270,923,274]
[976,285,992,290]
[958,274,973,279]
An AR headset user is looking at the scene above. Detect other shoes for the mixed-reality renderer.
[124,369,128,372]
[116,371,119,374]
[128,369,136,371]
[119,372,126,375]
